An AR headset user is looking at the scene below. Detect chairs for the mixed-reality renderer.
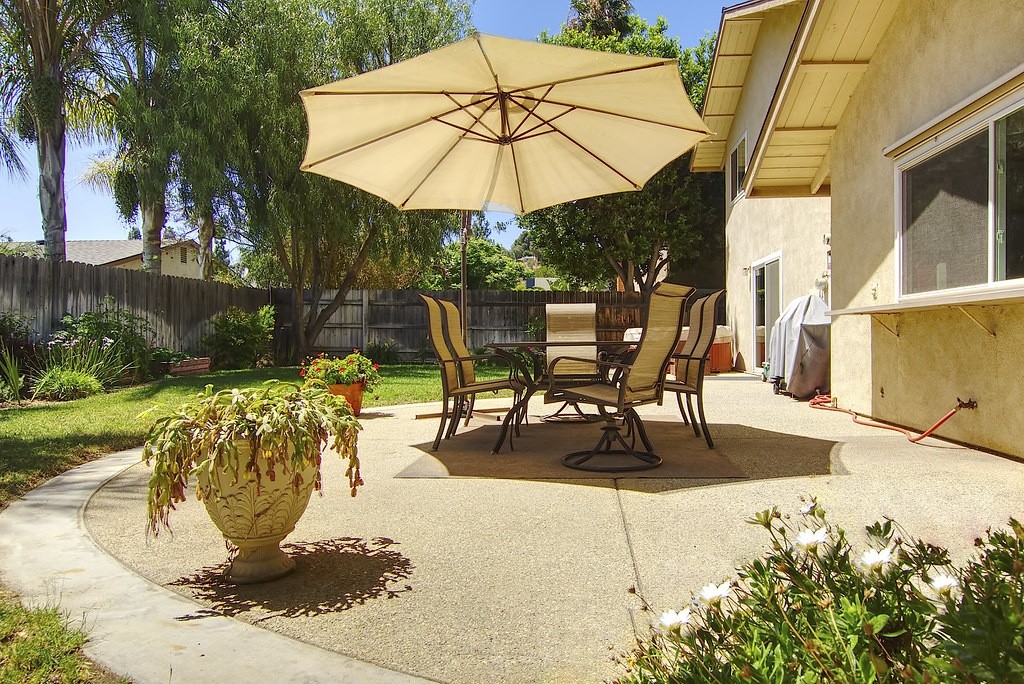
[627,288,728,451]
[613,295,709,427]
[419,293,525,453]
[437,299,529,436]
[542,281,697,475]
[526,302,610,426]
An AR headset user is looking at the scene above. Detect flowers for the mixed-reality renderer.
[300,348,386,401]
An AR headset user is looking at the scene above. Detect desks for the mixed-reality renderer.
[486,340,655,457]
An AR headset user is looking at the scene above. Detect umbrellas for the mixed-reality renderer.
[296,32,719,419]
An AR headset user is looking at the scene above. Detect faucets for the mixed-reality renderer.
[955,396,968,412]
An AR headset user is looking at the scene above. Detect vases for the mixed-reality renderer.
[324,382,366,416]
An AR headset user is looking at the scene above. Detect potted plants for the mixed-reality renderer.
[139,378,366,585]
